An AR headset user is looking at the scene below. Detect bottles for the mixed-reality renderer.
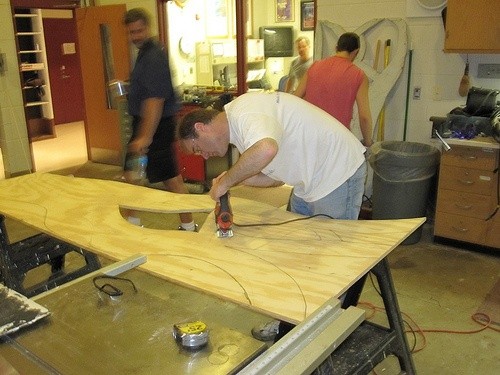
[137,155,148,179]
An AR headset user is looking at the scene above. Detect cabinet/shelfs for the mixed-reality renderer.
[171,103,208,185]
[119,99,134,176]
[444,0,500,55]
[195,36,265,89]
[12,8,57,142]
[432,135,500,257]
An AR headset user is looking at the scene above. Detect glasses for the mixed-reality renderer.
[193,135,203,156]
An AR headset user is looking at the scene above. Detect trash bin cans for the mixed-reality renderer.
[366,140,441,245]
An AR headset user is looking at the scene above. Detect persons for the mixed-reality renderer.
[294,32,374,147]
[286,36,313,93]
[123,7,197,232]
[179,91,367,342]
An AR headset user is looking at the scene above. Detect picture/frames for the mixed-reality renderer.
[274,0,295,23]
[300,0,315,31]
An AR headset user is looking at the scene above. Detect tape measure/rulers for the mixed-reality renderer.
[173,321,210,352]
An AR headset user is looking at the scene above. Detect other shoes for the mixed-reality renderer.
[176,224,198,232]
[251,318,280,341]
